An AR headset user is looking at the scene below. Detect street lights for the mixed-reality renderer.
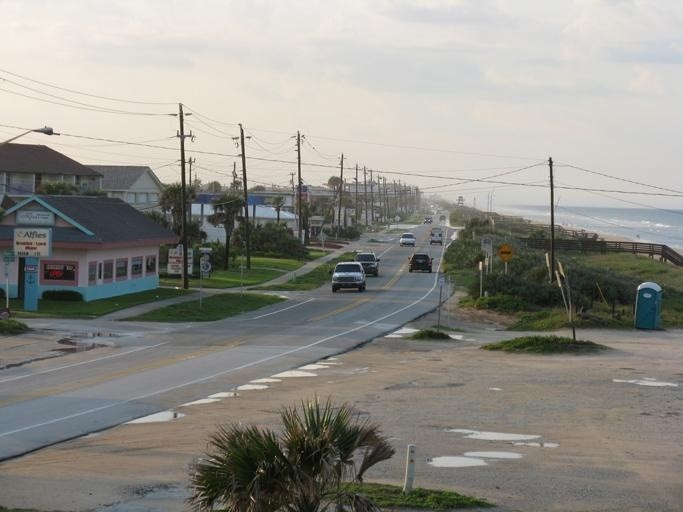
[0,127,53,148]
[343,177,357,241]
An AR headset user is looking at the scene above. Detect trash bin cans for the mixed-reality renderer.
[635,281,663,329]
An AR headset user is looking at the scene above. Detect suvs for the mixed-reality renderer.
[407,251,434,273]
[327,262,368,292]
[429,227,443,246]
[423,216,432,224]
[350,249,380,277]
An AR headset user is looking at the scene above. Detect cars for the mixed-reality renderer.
[399,233,416,247]
[421,198,441,214]
[439,216,446,222]
